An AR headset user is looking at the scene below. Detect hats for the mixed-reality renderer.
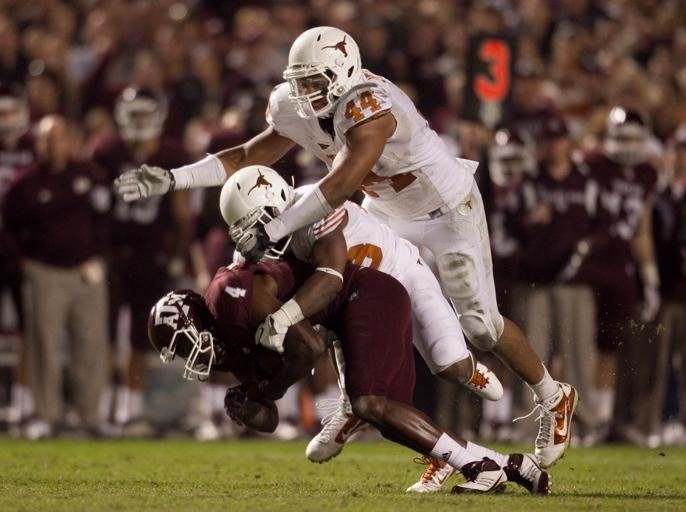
[539,115,567,139]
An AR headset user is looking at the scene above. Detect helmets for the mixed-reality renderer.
[147,288,222,384]
[0,82,30,145]
[488,126,533,189]
[603,103,651,166]
[219,164,298,264]
[281,26,361,120]
[113,81,170,143]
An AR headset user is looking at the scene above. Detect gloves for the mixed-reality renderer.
[641,286,660,323]
[114,163,170,204]
[236,226,270,264]
[255,311,288,353]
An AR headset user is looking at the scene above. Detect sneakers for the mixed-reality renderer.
[405,456,460,494]
[624,424,684,447]
[6,406,79,441]
[452,455,508,493]
[569,419,606,447]
[305,397,370,462]
[459,423,515,440]
[182,417,243,440]
[256,421,298,440]
[98,409,160,439]
[504,451,552,495]
[465,351,503,401]
[534,382,579,469]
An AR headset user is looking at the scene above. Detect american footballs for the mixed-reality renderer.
[266,324,327,366]
